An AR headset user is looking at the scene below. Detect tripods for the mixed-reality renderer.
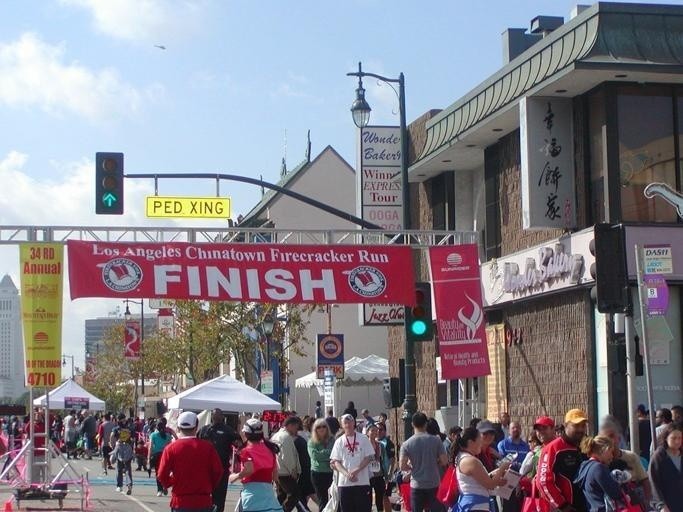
[0,414,21,480]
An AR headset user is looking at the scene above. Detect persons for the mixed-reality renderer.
[228,400,683,512]
[0,410,178,498]
[195,407,238,512]
[157,411,225,512]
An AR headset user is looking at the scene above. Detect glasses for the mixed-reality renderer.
[316,425,327,429]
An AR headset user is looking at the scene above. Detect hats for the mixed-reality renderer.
[564,409,588,425]
[533,417,553,429]
[477,421,496,434]
[242,419,262,434]
[177,411,196,429]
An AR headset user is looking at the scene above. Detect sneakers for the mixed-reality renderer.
[67,452,92,459]
[137,466,151,477]
[115,486,122,492]
[156,487,168,497]
[126,484,132,495]
[108,466,115,469]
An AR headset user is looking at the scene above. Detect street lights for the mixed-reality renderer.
[61,354,74,381]
[122,298,144,396]
[85,341,98,377]
[260,314,275,370]
[344,60,418,444]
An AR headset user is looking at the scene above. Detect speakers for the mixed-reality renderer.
[383,377,399,409]
[144,401,161,417]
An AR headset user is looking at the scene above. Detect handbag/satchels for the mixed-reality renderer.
[437,465,458,507]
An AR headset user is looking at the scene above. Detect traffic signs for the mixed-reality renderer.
[147,197,229,219]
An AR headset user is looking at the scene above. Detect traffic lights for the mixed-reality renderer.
[93,151,125,216]
[407,281,434,342]
[586,220,626,313]
